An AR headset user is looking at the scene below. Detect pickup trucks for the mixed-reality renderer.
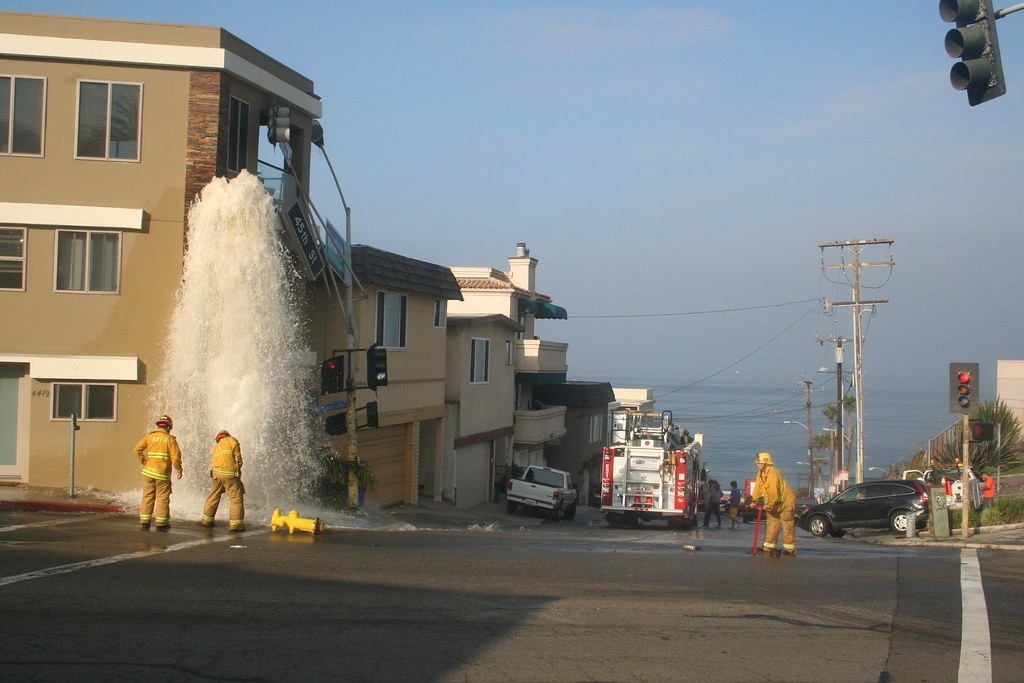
[505,465,578,523]
[902,467,985,515]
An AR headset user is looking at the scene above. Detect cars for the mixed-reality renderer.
[743,496,767,523]
[794,497,818,525]
[796,479,929,538]
[717,499,729,514]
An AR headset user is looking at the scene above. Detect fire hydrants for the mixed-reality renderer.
[271,508,324,536]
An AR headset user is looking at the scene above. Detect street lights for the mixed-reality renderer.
[796,461,822,487]
[309,117,356,381]
[783,420,815,499]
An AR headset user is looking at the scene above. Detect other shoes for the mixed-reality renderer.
[155,525,171,532]
[141,523,152,531]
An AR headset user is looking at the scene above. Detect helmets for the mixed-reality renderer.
[215,429,230,441]
[754,452,774,465]
[156,415,173,429]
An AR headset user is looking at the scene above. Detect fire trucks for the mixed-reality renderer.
[599,410,702,531]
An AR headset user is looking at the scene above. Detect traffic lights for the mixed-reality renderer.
[949,363,979,415]
[368,346,389,391]
[972,423,993,441]
[267,105,292,145]
[321,355,344,394]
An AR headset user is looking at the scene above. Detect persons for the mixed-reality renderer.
[727,480,741,529]
[133,415,183,533]
[980,471,996,509]
[749,452,797,556]
[703,479,723,528]
[199,430,246,532]
[925,480,937,526]
